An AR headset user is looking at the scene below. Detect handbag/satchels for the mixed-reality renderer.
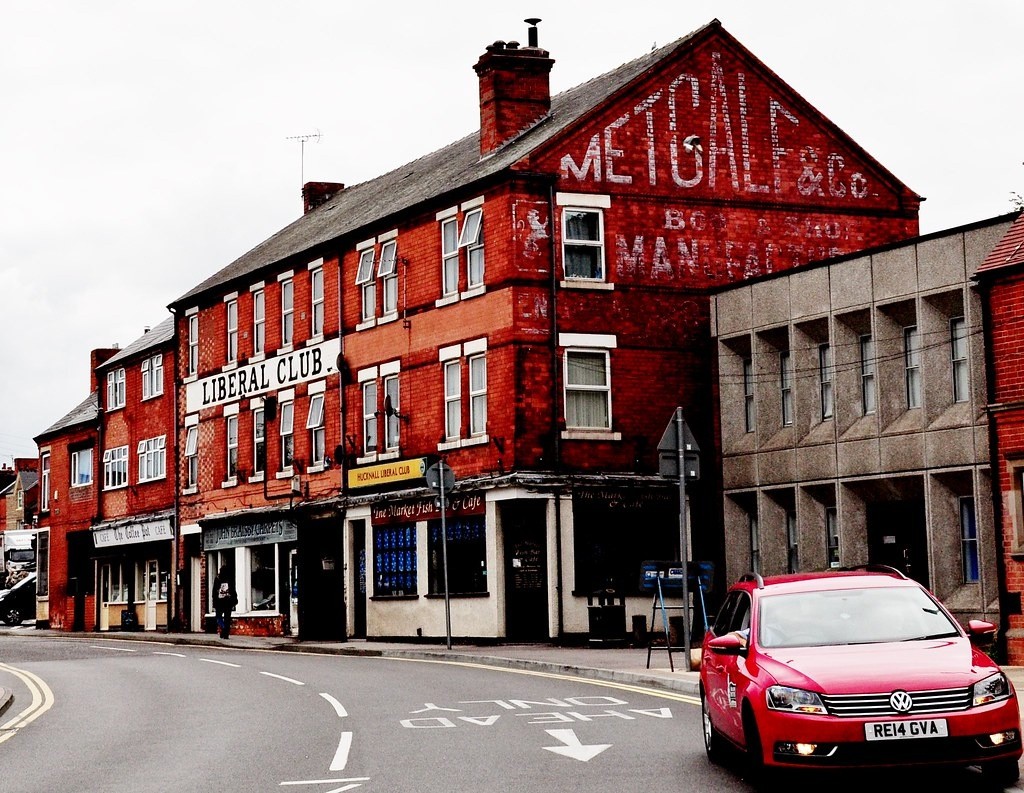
[225,587,238,606]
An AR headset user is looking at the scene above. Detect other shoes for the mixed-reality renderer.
[219,627,227,639]
[224,636,229,639]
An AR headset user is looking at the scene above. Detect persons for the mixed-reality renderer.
[212,565,238,640]
[725,623,787,647]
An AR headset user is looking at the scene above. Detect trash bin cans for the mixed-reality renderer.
[586,589,626,648]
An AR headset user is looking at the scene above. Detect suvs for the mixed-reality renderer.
[698,561,1022,791]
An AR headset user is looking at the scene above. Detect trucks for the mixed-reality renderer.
[0,530,37,575]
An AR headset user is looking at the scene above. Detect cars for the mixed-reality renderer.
[0,574,37,627]
